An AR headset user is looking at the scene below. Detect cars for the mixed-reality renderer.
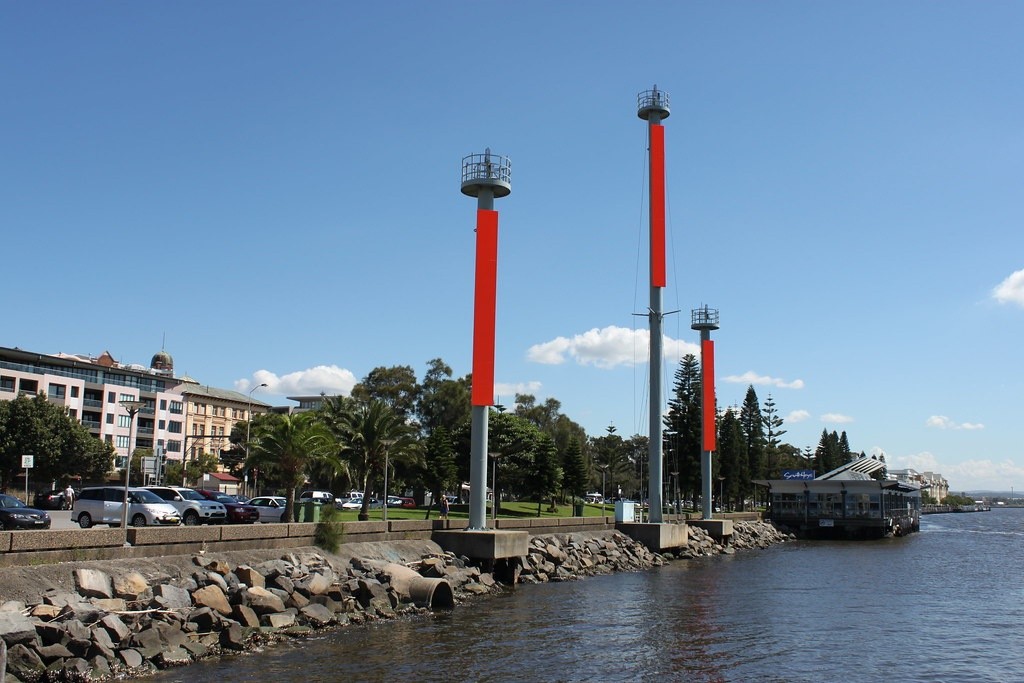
[229,493,251,505]
[192,487,260,525]
[245,495,290,523]
[0,494,52,531]
[33,490,80,510]
[604,496,701,508]
[342,497,384,511]
[335,492,365,504]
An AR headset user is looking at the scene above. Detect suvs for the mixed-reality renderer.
[70,486,184,529]
[136,486,228,526]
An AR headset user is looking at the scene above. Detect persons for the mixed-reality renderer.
[64,485,75,510]
[441,494,449,519]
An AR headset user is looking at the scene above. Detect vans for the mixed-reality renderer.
[444,495,465,504]
[386,497,418,509]
[299,490,335,503]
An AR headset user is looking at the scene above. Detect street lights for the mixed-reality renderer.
[244,383,269,488]
[117,399,148,540]
